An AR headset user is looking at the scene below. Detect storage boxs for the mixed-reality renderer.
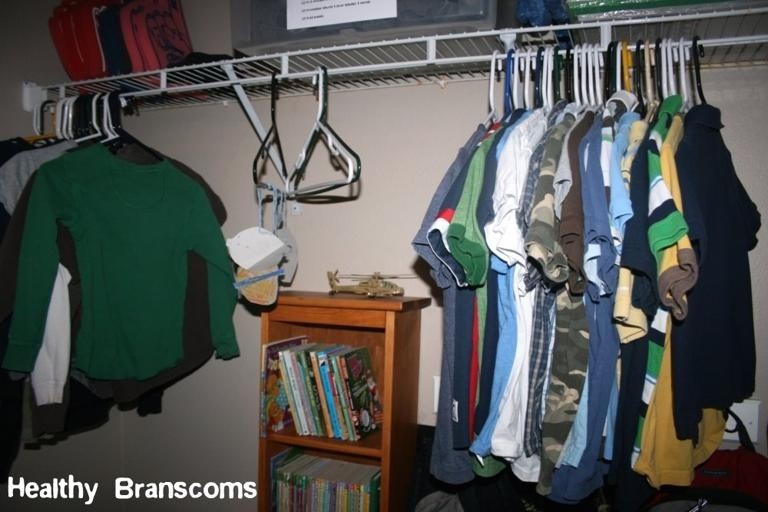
[230,1,500,75]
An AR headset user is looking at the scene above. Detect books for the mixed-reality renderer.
[268,446,381,512]
[258,334,382,444]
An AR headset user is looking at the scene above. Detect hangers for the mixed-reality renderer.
[251,66,360,197]
[474,37,709,136]
[16,92,167,165]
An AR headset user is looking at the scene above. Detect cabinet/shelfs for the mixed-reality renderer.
[257,291,432,511]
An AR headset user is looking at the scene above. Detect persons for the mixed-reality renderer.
[326,268,418,299]
